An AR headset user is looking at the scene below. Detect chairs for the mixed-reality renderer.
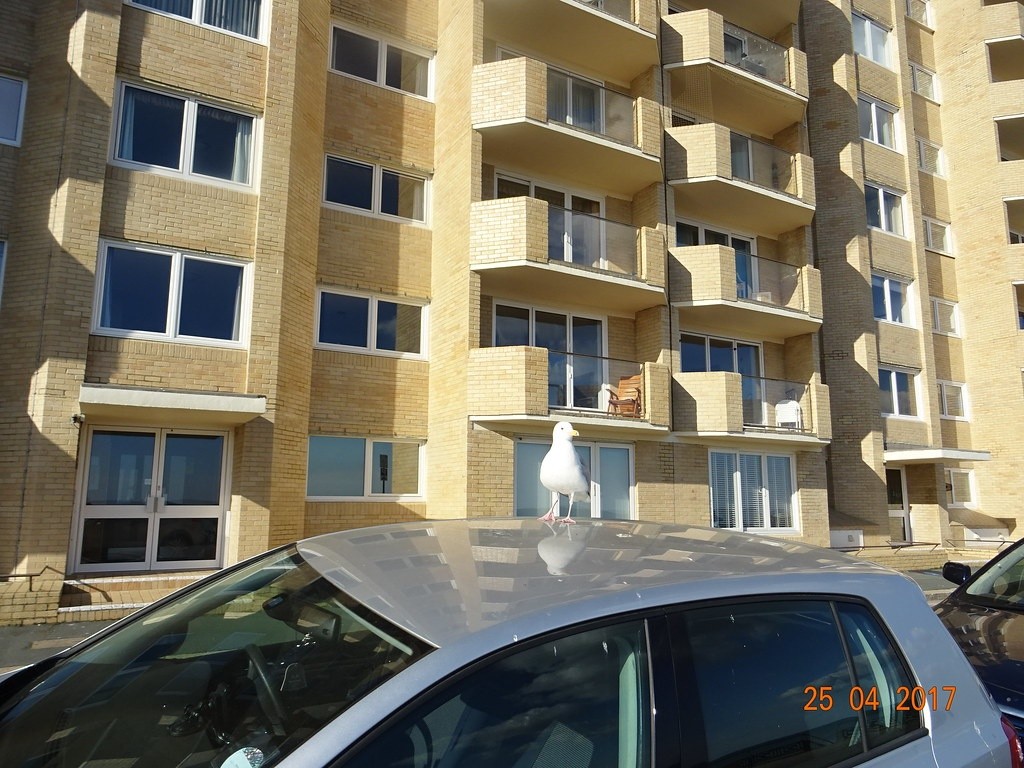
[407,656,522,768]
[605,375,643,418]
[512,635,618,768]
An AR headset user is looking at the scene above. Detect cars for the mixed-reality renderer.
[1,517,1024,768]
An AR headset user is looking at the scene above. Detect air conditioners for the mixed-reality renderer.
[742,62,769,76]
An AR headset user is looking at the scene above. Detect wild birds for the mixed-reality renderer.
[536,420,593,523]
